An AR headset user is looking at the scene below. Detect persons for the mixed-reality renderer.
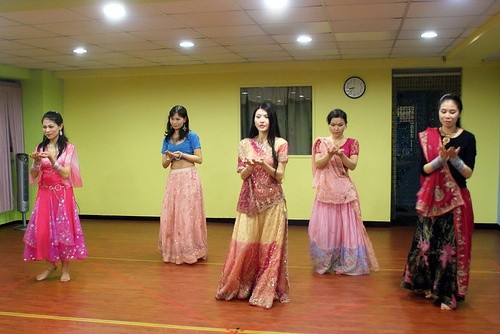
[307,108,379,277]
[400,93,477,311]
[157,104,208,265]
[214,101,291,311]
[22,111,88,283]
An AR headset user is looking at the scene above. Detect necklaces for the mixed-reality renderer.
[170,136,180,144]
[47,142,56,150]
[439,127,458,145]
[332,138,343,147]
